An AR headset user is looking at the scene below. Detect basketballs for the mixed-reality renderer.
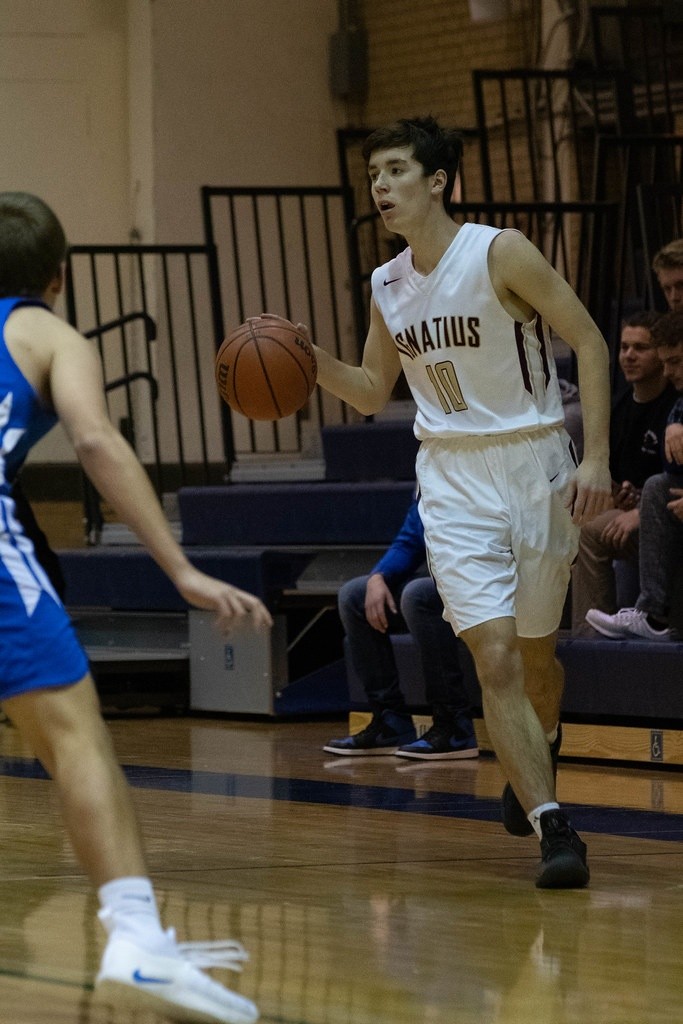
[215,317,316,424]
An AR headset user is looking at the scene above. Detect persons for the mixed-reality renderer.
[323,500,480,759]
[0,190,272,1024]
[559,240,683,642]
[247,113,611,889]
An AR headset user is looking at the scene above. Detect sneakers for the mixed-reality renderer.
[586,605,673,643]
[394,715,480,760]
[321,708,418,755]
[499,720,562,837]
[536,808,590,889]
[93,935,260,1024]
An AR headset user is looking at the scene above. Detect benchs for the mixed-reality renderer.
[52,340,682,730]
